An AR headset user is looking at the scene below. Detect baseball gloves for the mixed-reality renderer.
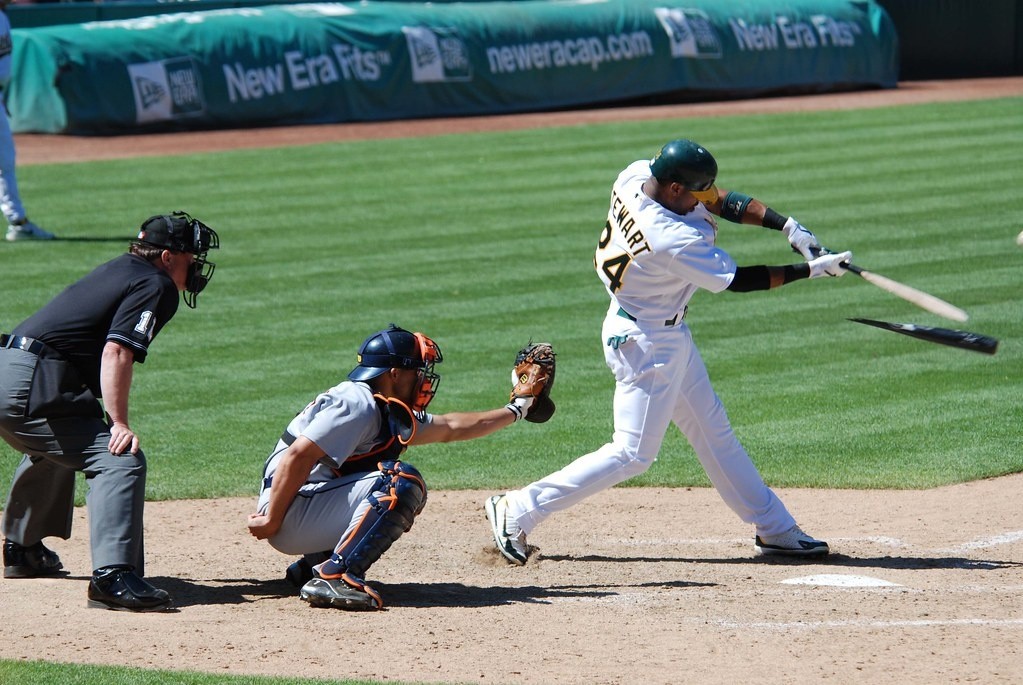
[508,342,557,424]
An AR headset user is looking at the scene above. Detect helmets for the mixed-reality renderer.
[347,325,421,382]
[649,138,719,192]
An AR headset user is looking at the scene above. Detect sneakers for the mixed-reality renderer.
[300,575,379,611]
[754,524,829,553]
[5,220,56,241]
[483,494,530,566]
[285,558,316,586]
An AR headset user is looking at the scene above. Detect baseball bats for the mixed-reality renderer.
[790,242,971,323]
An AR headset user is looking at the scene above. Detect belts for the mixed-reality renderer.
[0,334,46,357]
[263,478,272,488]
[617,307,676,325]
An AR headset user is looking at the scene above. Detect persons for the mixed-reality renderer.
[247,326,555,609]
[0,211,220,610]
[485,138,852,565]
[0,10,54,240]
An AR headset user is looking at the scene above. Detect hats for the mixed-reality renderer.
[135,212,202,255]
[688,184,719,205]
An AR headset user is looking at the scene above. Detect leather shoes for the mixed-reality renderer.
[87,568,174,613]
[3,537,62,578]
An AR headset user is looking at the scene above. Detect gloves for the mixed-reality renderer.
[607,336,629,349]
[782,217,827,260]
[807,249,853,280]
